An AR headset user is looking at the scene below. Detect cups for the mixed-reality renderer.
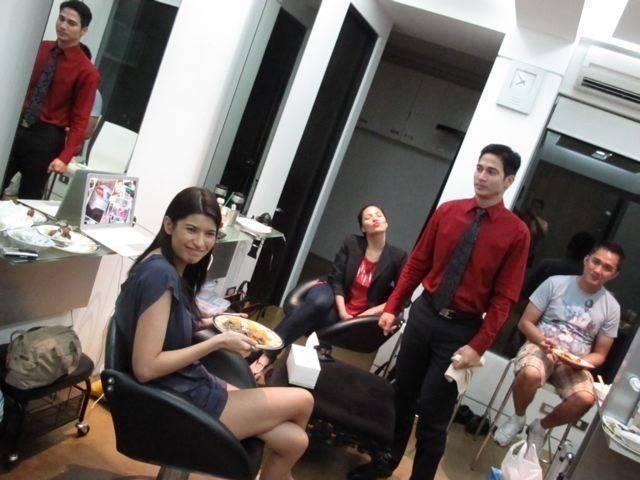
[212,183,229,210]
[109,193,131,224]
[227,192,245,225]
[543,323,559,352]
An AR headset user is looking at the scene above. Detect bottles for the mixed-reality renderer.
[84,181,115,223]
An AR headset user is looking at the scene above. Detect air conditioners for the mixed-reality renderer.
[572,44,640,99]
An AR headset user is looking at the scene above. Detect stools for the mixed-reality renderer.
[0,341,95,464]
[473,355,577,480]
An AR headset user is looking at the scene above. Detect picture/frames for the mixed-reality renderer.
[79,173,140,230]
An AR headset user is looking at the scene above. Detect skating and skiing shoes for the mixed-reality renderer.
[526,418,548,455]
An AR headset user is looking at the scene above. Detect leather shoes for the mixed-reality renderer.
[345,459,398,479]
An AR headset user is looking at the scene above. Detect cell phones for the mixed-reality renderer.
[3,246,39,260]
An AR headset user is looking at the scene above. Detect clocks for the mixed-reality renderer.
[495,60,547,116]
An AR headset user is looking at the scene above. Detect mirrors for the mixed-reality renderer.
[0,0,322,237]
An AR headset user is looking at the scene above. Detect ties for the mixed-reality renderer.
[22,45,64,125]
[428,207,489,313]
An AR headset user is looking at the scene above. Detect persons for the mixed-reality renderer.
[246,204,409,385]
[0,0,102,201]
[113,185,316,480]
[489,241,625,463]
[341,144,532,480]
[519,229,595,302]
[516,195,549,261]
[4,41,104,196]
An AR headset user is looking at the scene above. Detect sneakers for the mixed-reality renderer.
[455,403,497,434]
[493,414,526,448]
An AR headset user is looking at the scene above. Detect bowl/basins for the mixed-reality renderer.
[619,428,640,451]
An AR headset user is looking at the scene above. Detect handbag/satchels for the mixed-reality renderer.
[2,320,84,392]
[500,440,543,480]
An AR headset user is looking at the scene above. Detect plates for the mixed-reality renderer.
[600,413,629,438]
[555,348,596,371]
[37,223,98,255]
[211,313,285,351]
[38,225,71,245]
[6,228,55,254]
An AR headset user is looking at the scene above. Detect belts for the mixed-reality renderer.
[422,286,482,328]
[17,114,61,135]
